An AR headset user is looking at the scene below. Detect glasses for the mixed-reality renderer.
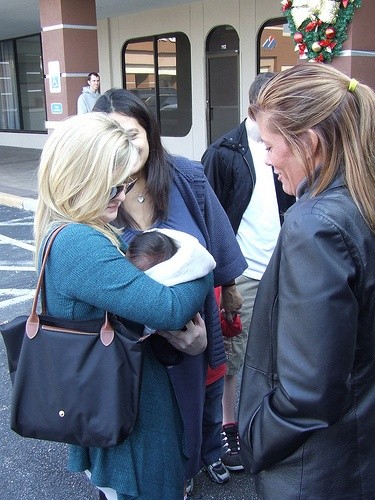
[109,176,138,200]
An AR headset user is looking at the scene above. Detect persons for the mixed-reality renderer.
[76,73,101,113]
[126,227,217,341]
[237,62,375,499]
[9,112,215,500]
[200,73,298,470]
[91,87,249,485]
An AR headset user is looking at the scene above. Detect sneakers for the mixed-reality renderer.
[204,458,230,484]
[186,478,193,495]
[219,423,245,471]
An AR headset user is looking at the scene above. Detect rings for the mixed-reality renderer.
[175,345,179,348]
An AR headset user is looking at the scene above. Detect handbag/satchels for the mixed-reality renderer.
[0,223,151,448]
[214,286,242,337]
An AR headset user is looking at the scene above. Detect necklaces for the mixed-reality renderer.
[129,184,147,202]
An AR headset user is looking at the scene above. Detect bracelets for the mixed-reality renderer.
[221,282,236,287]
